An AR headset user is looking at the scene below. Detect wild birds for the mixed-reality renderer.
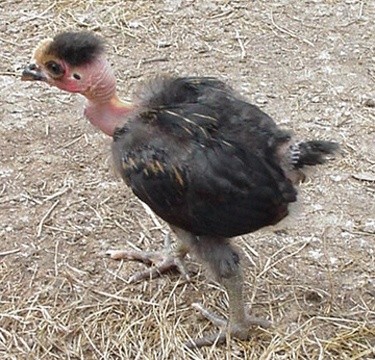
[20,28,349,348]
